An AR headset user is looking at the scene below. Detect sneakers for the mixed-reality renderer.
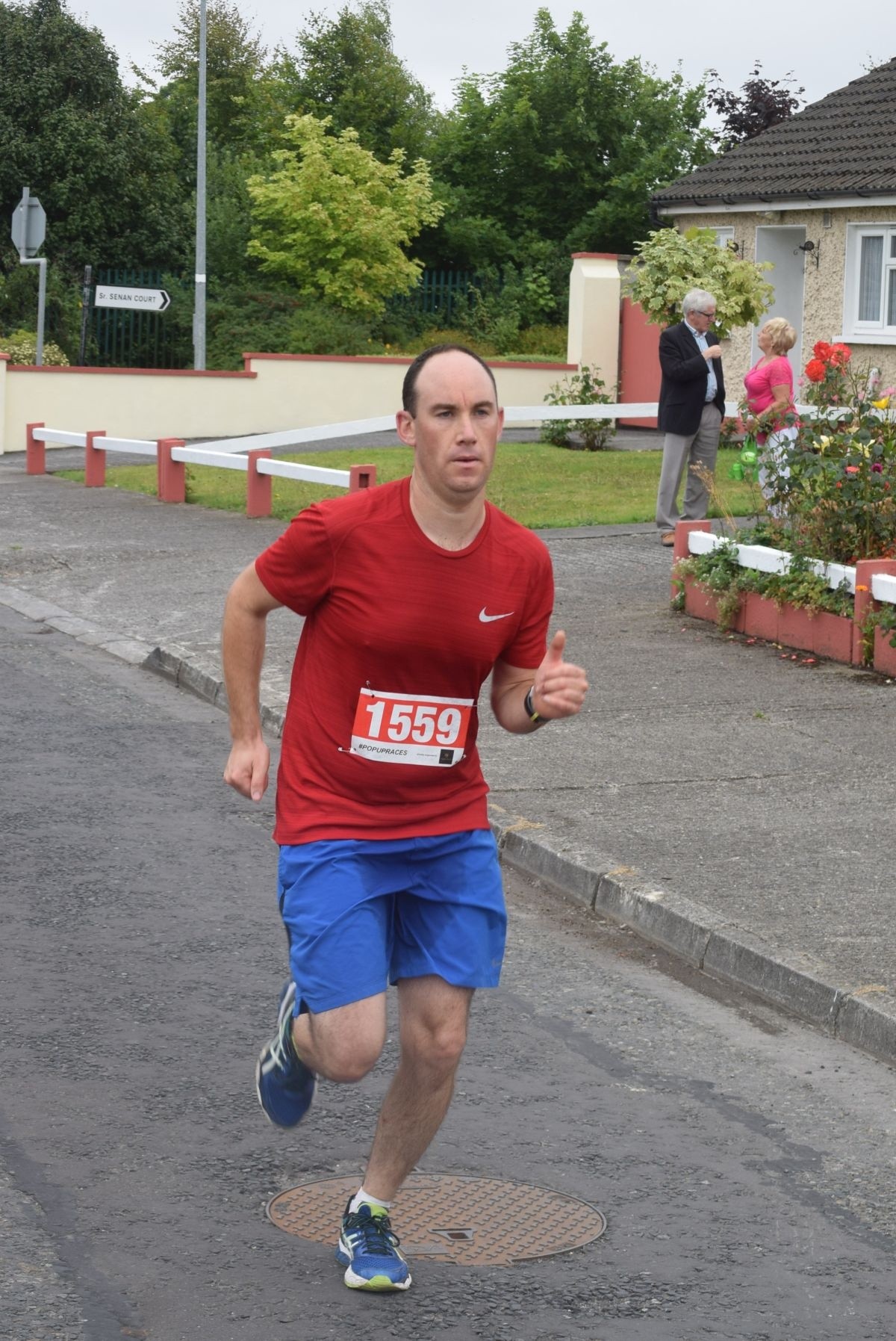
[259,978,322,1129]
[337,1193,414,1292]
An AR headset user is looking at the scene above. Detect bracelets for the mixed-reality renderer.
[523,684,553,724]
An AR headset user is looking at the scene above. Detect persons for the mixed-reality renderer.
[655,289,727,545]
[222,344,588,1290]
[744,317,799,537]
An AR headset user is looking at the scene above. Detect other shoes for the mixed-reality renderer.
[662,532,674,546]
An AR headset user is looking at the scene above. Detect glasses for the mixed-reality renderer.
[694,311,715,320]
